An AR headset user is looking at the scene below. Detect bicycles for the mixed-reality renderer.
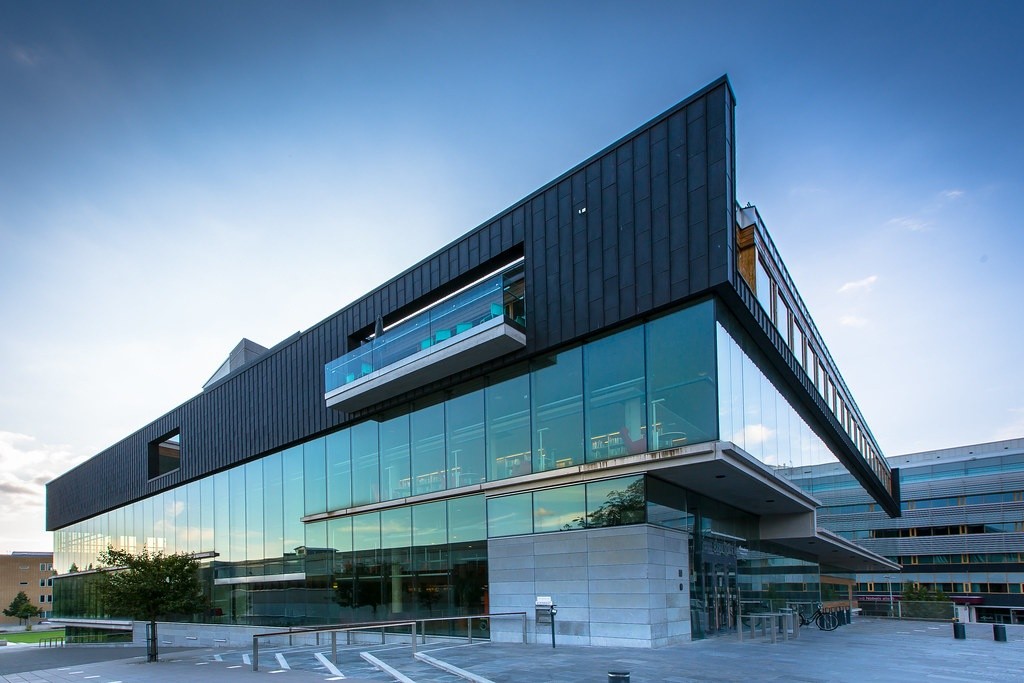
[795,602,839,631]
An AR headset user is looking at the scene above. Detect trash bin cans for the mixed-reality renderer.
[954,622,966,639]
[993,625,1007,642]
[607,670,630,683]
